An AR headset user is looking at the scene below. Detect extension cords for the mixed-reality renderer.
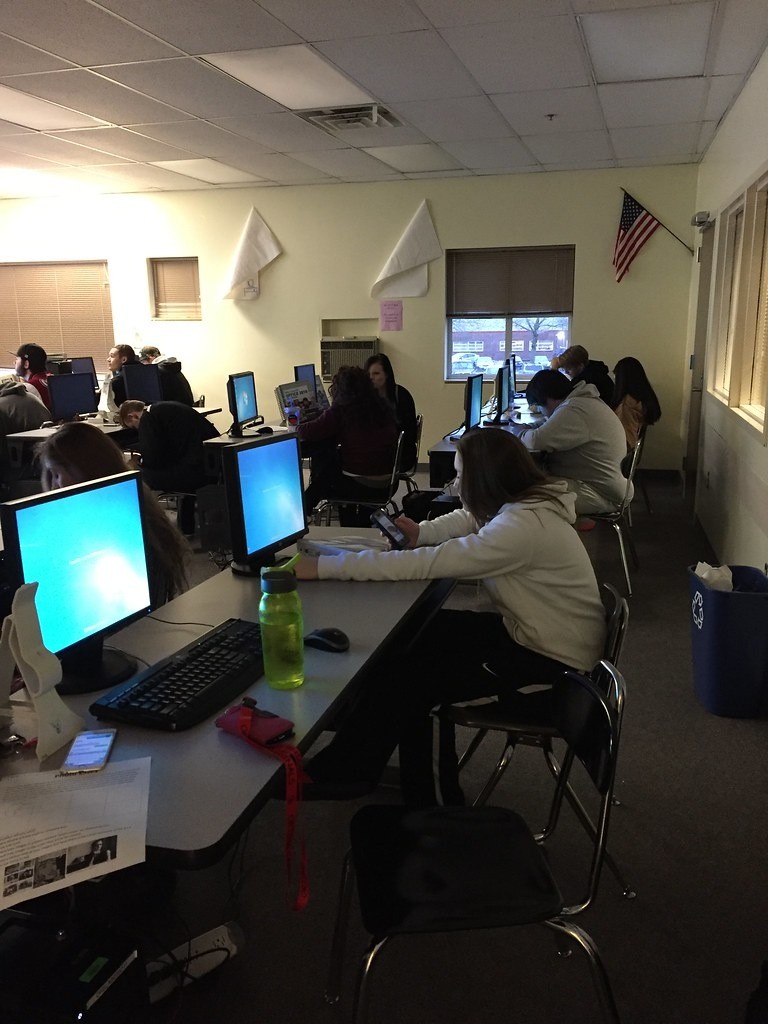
[146,921,248,1003]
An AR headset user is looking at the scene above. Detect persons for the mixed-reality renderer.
[295,427,609,798]
[0,345,662,625]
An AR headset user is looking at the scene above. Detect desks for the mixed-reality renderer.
[428,385,564,489]
[0,521,450,874]
[5,403,223,481]
[201,415,313,452]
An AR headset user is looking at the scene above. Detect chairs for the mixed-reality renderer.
[0,369,655,1024]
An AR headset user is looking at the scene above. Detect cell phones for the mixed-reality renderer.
[370,510,409,549]
[60,728,117,774]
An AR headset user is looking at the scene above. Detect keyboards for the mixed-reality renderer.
[88,617,264,732]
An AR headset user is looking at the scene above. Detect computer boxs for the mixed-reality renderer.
[0,908,152,1024]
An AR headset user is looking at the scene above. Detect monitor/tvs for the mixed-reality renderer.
[294,364,317,403]
[461,353,521,438]
[227,370,261,438]
[0,469,157,694]
[121,364,163,402]
[66,357,99,390]
[221,431,309,577]
[46,372,98,425]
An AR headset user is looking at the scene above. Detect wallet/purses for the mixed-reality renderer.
[215,698,296,749]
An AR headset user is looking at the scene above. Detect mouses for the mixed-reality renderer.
[304,627,350,653]
[256,426,273,433]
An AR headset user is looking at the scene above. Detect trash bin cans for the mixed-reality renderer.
[687,564,768,719]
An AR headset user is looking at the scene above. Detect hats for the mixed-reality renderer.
[7,342,46,363]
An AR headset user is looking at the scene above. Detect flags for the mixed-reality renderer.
[612,192,659,284]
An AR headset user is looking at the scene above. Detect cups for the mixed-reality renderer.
[283,406,300,433]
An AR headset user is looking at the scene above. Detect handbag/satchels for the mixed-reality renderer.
[197,446,235,556]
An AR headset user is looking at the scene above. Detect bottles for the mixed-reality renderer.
[258,566,305,692]
